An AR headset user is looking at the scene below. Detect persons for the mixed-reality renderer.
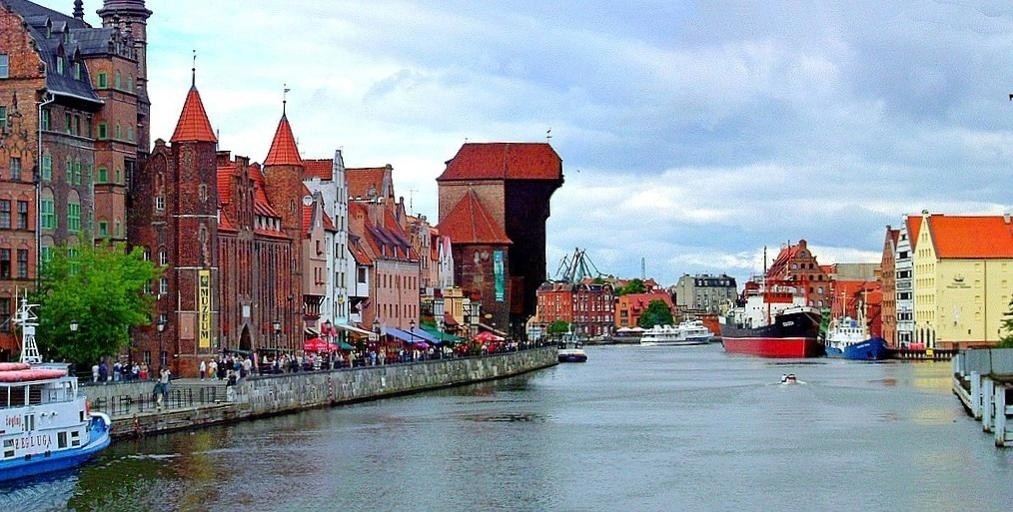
[781,374,787,382]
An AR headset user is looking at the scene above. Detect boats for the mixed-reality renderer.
[0,475,82,512]
[0,277,115,481]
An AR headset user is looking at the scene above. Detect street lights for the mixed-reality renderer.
[154,318,169,379]
[407,316,417,364]
[271,319,282,376]
[322,316,332,371]
[69,318,81,381]
[430,316,555,359]
[370,318,382,367]
